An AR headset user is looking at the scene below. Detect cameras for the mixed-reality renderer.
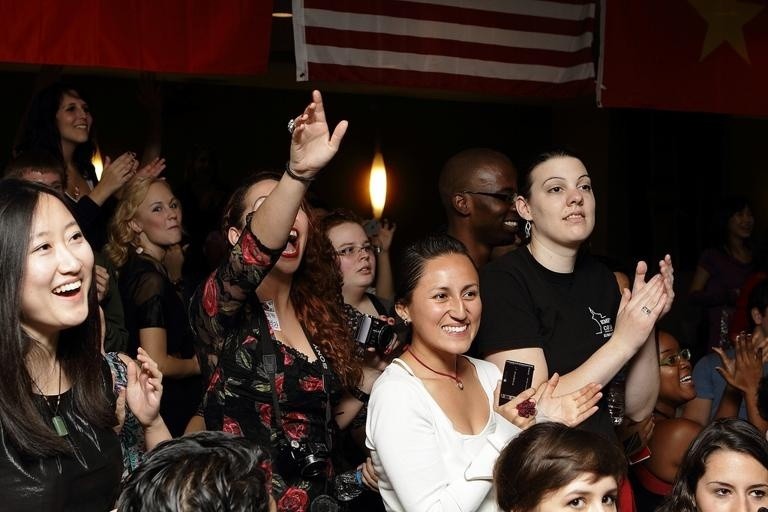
[363,220,378,237]
[272,437,329,480]
[356,314,409,357]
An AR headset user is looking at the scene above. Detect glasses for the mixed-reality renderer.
[336,245,375,257]
[659,348,691,368]
[464,190,518,206]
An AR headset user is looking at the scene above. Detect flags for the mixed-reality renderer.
[590,1,768,118]
[0,2,269,77]
[288,0,597,99]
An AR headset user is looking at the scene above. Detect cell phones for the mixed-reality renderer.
[499,360,534,405]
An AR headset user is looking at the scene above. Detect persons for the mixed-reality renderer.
[2,83,768,510]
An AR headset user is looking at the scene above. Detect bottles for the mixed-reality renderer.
[336,470,363,501]
[607,383,626,426]
